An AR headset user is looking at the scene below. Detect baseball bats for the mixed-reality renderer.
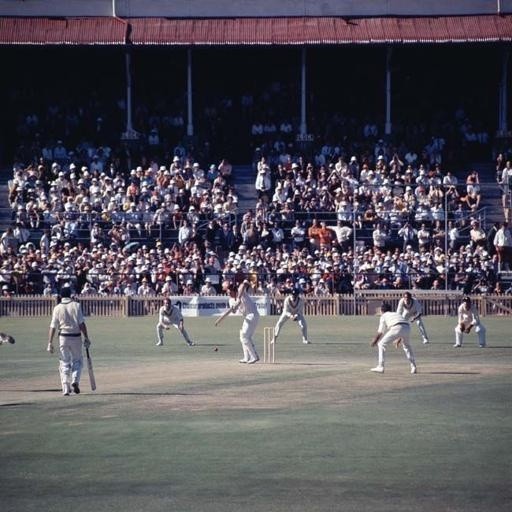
[86,348,95,390]
[215,309,232,326]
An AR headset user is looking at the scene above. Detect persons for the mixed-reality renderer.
[370,304,417,373]
[270,288,310,344]
[226,278,260,364]
[0,117,512,297]
[46,288,91,395]
[392,292,429,345]
[453,296,486,348]
[156,297,196,346]
[1,332,15,345]
